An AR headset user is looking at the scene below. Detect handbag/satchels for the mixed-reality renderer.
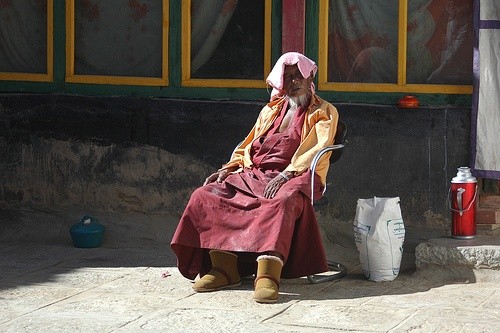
[353,196,405,283]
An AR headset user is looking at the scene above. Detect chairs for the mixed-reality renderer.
[307,120,348,284]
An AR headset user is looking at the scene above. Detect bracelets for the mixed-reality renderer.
[279,172,289,181]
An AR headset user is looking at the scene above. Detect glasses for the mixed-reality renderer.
[284,69,302,83]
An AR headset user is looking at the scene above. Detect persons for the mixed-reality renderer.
[170,52,339,303]
[332,0,476,85]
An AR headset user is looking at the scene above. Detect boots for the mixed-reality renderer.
[253,255,284,303]
[192,250,241,292]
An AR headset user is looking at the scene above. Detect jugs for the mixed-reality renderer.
[446,166,478,238]
